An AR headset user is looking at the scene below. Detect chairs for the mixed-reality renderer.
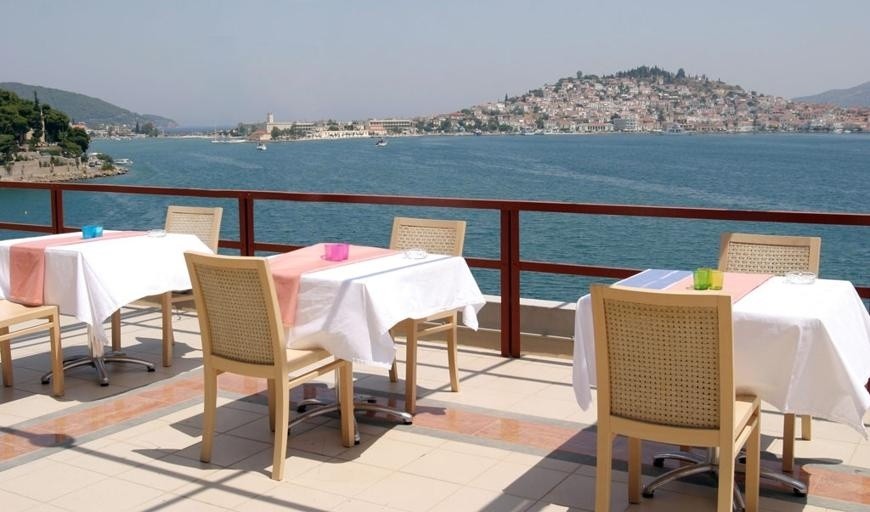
[0,299,65,397]
[386,216,467,414]
[184,252,355,482]
[112,206,224,366]
[589,283,761,512]
[678,233,821,475]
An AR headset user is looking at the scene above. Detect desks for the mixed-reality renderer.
[265,244,486,445]
[573,269,870,512]
[0,229,215,387]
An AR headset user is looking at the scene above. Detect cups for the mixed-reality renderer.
[81,222,104,238]
[692,266,724,290]
[322,236,350,261]
